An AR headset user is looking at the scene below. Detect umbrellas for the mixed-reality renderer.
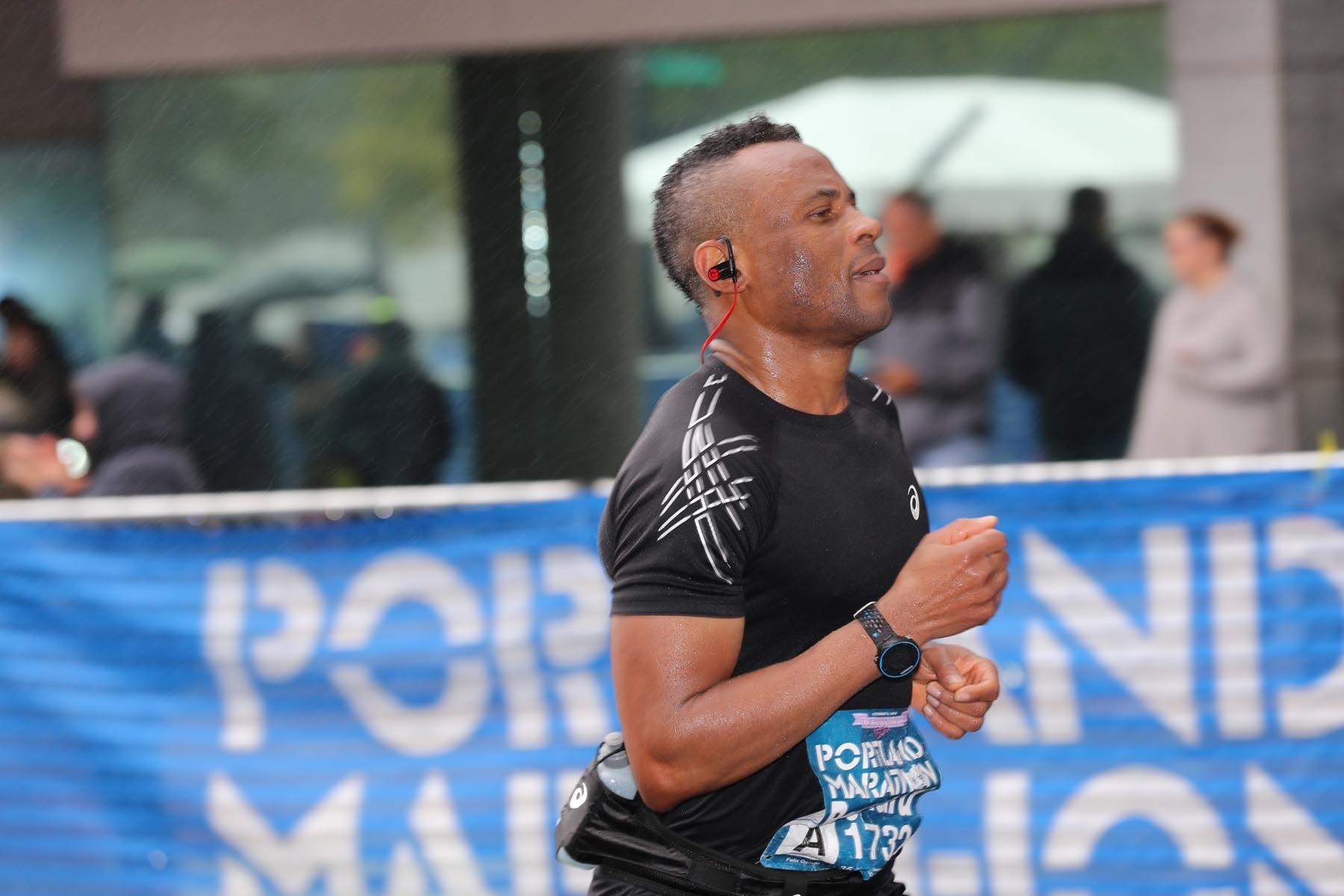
[615,76,1187,243]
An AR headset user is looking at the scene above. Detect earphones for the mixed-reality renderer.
[706,260,735,282]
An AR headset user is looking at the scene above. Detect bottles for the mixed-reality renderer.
[596,731,637,800]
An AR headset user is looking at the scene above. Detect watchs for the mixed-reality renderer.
[851,601,922,679]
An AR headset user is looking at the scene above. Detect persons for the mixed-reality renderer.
[0,289,465,502]
[552,115,1010,896]
[850,180,1302,468]
